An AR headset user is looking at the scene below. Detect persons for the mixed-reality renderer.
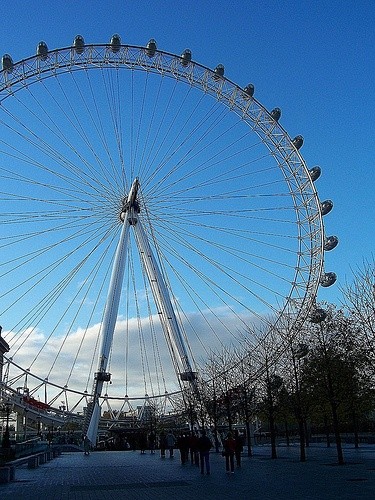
[224,431,243,473]
[130,431,156,454]
[159,429,176,456]
[47,431,51,441]
[179,430,210,475]
[84,436,89,455]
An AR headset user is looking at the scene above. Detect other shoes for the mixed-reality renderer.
[225,471,234,474]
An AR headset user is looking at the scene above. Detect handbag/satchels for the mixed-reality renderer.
[222,450,225,457]
